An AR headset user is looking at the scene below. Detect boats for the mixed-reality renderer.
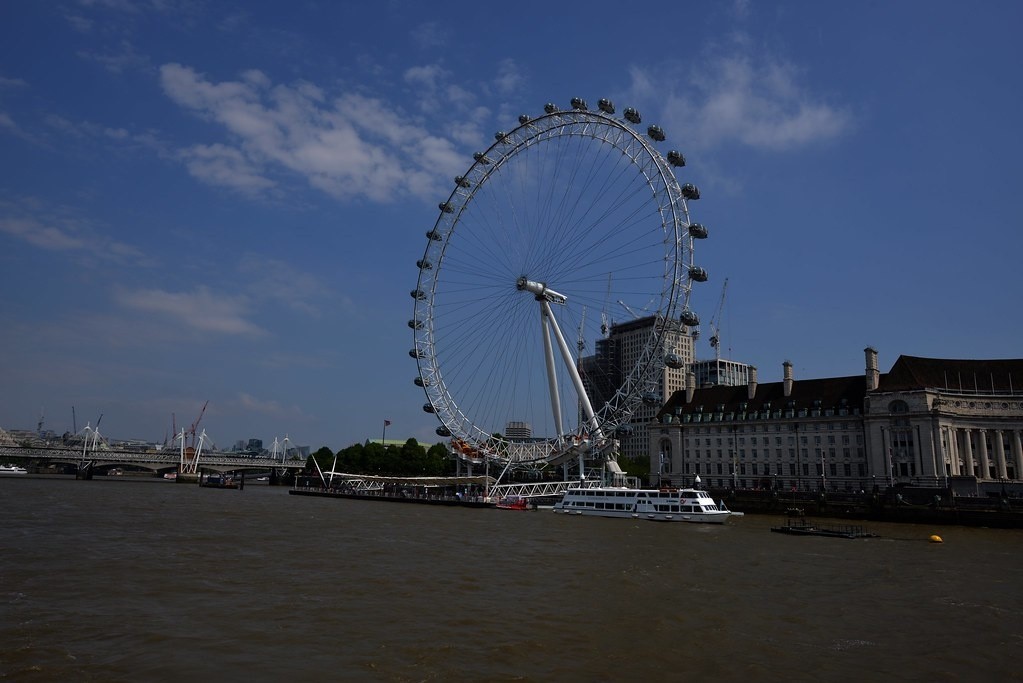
[0,464,28,479]
[202,471,240,492]
[552,471,745,525]
[495,493,530,511]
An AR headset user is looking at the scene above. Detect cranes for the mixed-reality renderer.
[573,271,643,374]
[708,277,731,360]
[168,399,212,458]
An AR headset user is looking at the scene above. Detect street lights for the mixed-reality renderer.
[382,419,394,447]
